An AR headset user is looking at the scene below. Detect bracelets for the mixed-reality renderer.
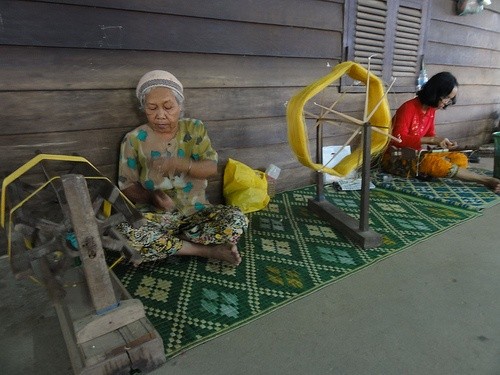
[185,157,194,176]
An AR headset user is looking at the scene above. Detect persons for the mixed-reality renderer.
[111,70,249,266]
[380,72,500,194]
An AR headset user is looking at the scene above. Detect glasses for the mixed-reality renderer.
[439,98,454,104]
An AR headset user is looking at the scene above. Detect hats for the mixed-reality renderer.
[136,70,183,103]
